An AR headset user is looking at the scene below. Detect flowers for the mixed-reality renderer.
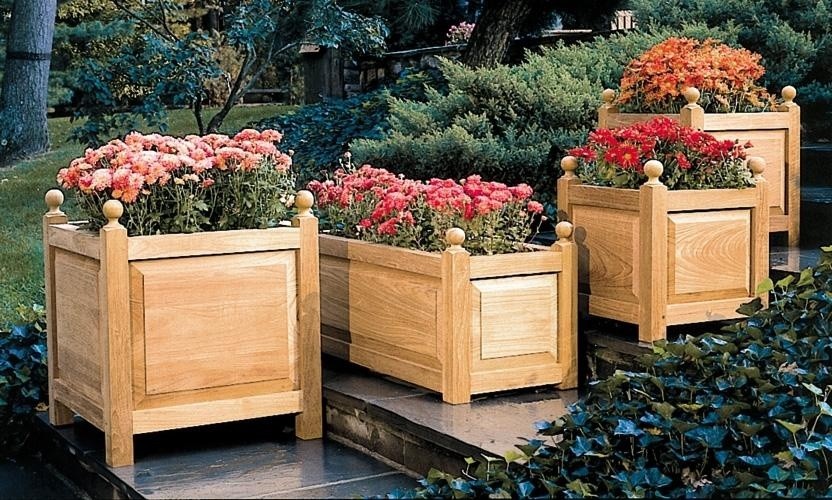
[563,35,779,190]
[57,126,547,257]
